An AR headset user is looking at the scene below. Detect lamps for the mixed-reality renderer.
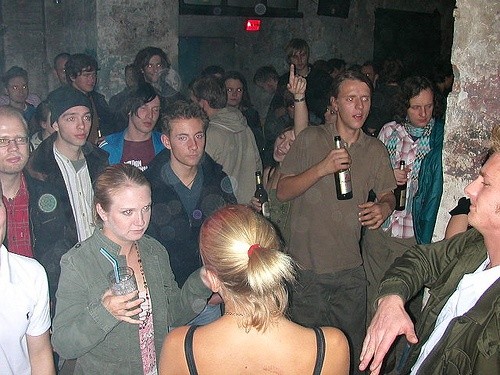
[254,0,269,15]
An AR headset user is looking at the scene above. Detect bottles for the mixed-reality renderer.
[395,160,407,210]
[97,127,102,147]
[334,136,353,200]
[254,171,271,219]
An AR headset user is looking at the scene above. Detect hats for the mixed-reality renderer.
[50,87,92,127]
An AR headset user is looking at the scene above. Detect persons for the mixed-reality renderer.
[0,39,499,375]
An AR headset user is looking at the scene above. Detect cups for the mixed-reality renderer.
[105,266,139,310]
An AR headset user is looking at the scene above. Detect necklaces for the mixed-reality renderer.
[134,241,151,330]
[297,66,312,78]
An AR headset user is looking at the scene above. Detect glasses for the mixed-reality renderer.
[0,136,29,147]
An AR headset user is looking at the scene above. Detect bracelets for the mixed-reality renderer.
[295,96,304,102]
